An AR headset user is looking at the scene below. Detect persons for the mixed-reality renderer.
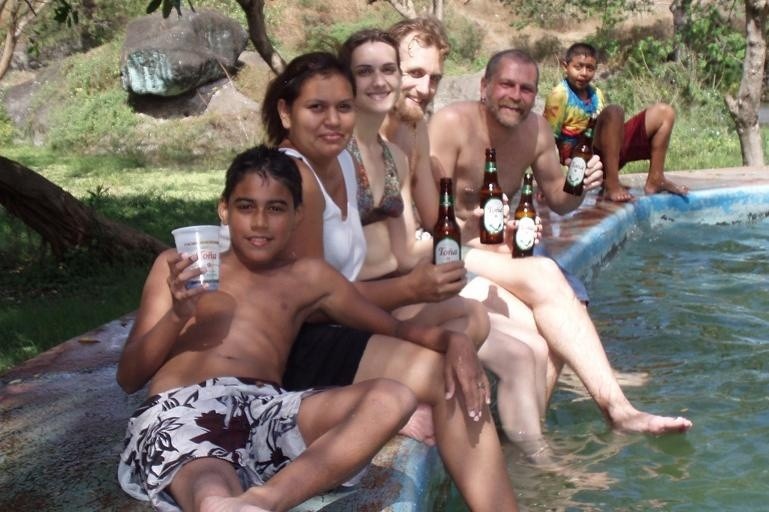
[424,48,650,392]
[261,52,523,512]
[335,31,582,482]
[117,141,493,511]
[542,41,689,203]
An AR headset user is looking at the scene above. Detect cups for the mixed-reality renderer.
[172,226,223,290]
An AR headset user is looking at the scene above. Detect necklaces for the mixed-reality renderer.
[386,16,694,435]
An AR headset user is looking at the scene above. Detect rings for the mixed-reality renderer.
[477,381,484,388]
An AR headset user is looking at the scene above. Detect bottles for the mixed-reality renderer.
[513,168,538,258]
[562,115,596,196]
[433,176,463,270]
[478,143,505,244]
